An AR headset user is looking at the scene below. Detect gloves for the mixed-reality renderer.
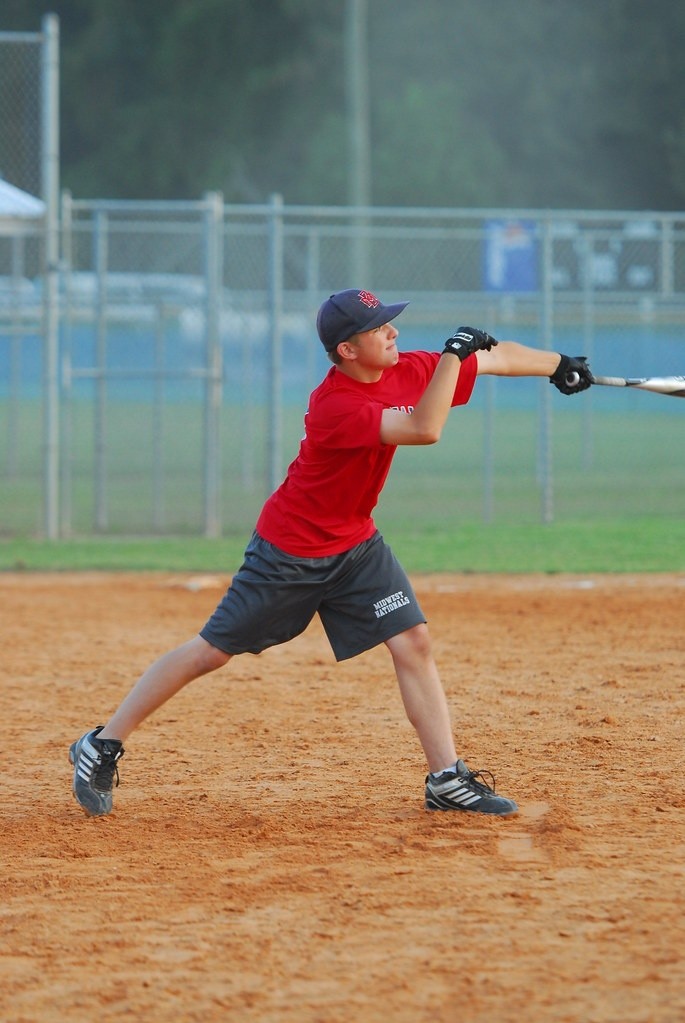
[441,327,498,364]
[548,354,593,395]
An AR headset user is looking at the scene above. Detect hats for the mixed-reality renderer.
[315,289,410,350]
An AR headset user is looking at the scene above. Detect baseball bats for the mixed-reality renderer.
[565,370,685,398]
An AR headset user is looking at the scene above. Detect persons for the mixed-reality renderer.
[68,287,597,815]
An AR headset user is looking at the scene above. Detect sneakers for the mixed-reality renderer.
[67,726,126,818]
[425,758,517,815]
